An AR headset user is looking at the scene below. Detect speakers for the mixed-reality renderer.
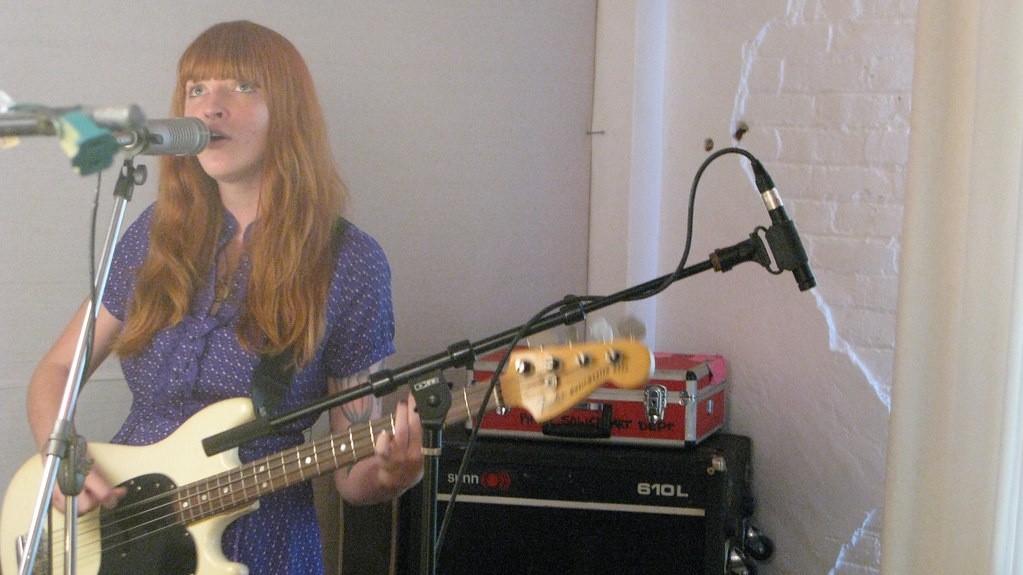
[427,424,752,575]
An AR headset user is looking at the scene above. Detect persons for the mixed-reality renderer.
[25,20,425,575]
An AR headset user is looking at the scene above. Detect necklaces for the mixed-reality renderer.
[215,247,233,301]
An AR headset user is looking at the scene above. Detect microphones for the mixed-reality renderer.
[750,160,816,293]
[117,116,211,156]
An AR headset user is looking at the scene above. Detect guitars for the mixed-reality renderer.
[1,302,655,575]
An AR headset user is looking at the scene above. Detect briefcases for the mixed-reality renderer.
[465,340,729,447]
